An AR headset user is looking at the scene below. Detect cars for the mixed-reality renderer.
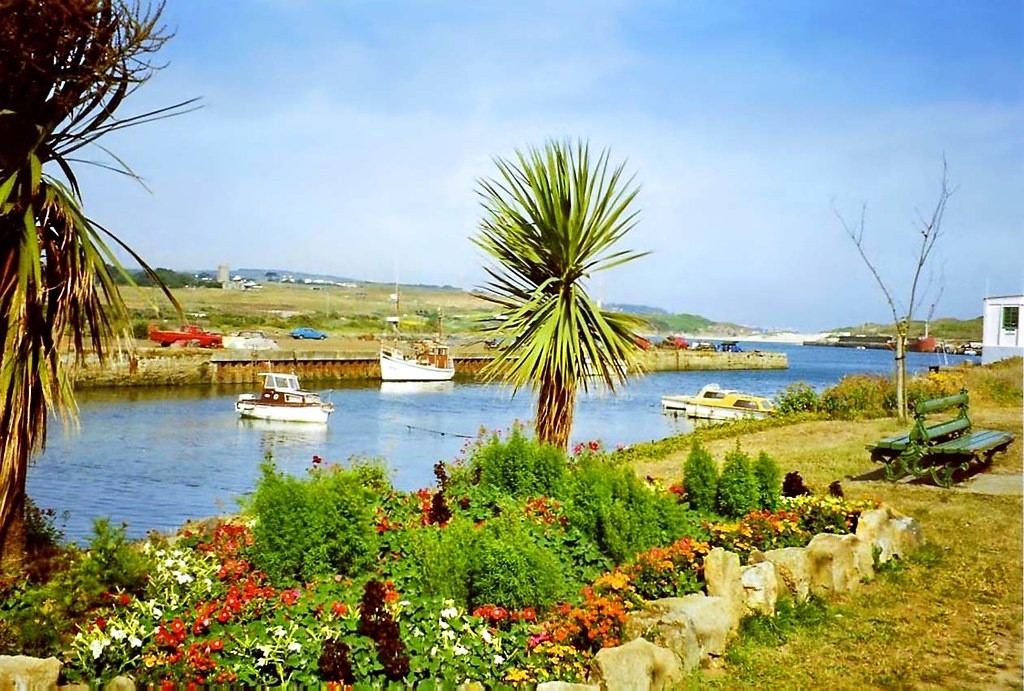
[289,327,328,340]
[484,337,510,351]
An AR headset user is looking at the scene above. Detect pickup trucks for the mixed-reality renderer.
[222,330,276,350]
[150,325,223,349]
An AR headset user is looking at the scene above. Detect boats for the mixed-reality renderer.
[234,357,335,424]
[379,264,455,381]
[661,383,776,420]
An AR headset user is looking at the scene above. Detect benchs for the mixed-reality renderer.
[866,387,968,482]
[918,389,1016,489]
